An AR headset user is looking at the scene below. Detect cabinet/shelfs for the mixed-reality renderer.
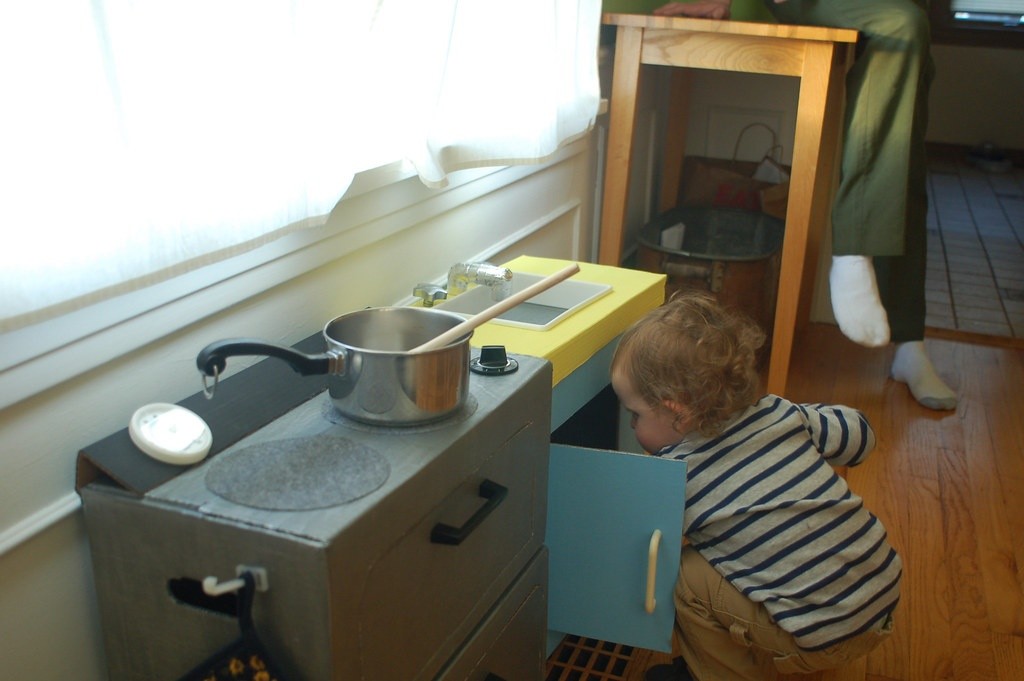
[544,328,690,670]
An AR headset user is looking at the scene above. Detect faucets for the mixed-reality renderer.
[411,259,514,308]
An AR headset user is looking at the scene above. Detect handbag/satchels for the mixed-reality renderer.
[679,123,776,212]
[751,145,790,220]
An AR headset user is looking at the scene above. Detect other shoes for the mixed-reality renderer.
[644,656,695,681]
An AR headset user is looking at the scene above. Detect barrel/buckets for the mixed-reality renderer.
[632,204,784,364]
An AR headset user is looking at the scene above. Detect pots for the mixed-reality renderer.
[196,305,473,425]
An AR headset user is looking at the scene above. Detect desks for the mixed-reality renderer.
[598,12,865,404]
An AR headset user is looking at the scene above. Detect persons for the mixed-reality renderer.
[609,289,903,681]
[653,0,959,411]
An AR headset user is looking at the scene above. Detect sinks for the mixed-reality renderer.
[428,270,613,331]
